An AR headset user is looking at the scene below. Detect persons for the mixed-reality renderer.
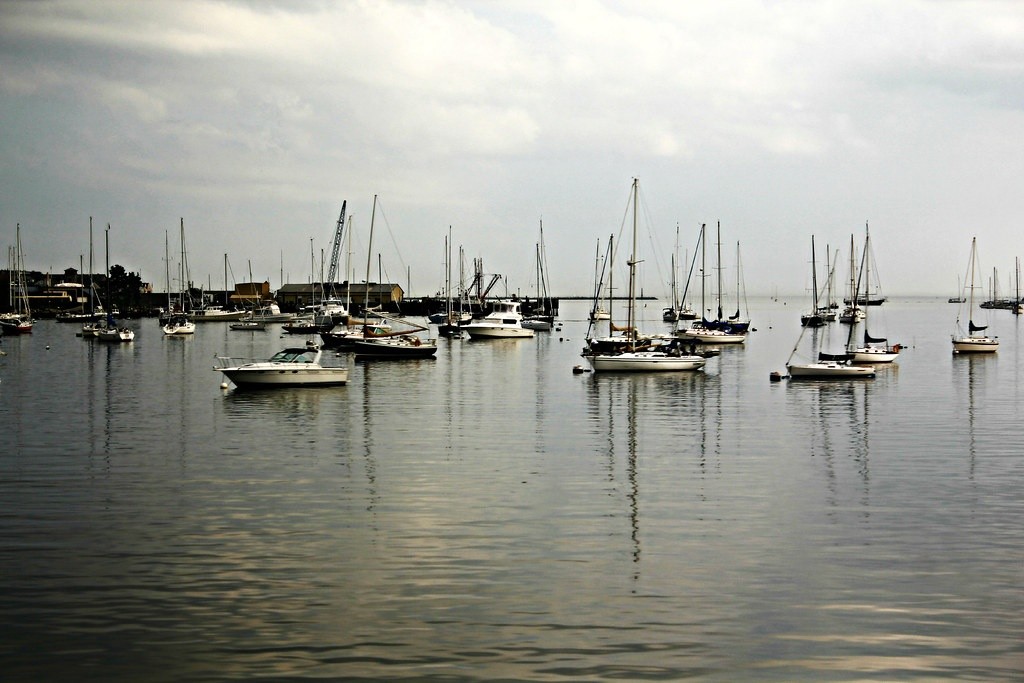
[690,342,696,356]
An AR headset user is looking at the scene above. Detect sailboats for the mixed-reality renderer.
[580,174,752,375]
[951,237,1000,353]
[779,218,907,378]
[949,257,1024,315]
[0,193,556,360]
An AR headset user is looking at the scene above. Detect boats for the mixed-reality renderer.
[210,339,355,390]
